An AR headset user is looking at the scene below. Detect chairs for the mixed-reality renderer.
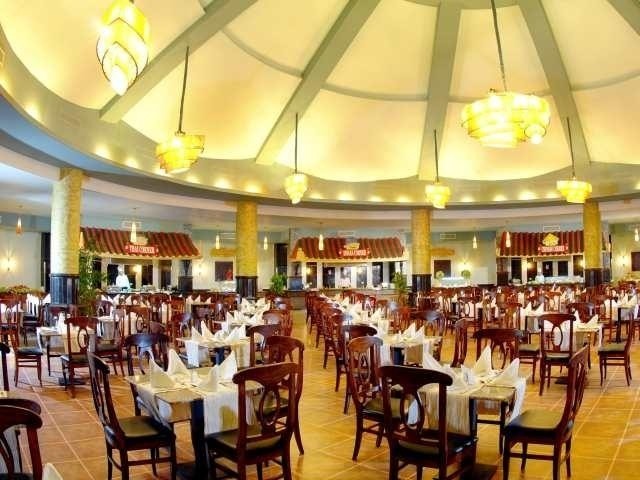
[347,336,417,460]
[205,363,298,479]
[252,337,304,465]
[86,350,177,480]
[503,346,589,479]
[380,366,478,480]
[0,406,42,478]
[0,397,41,414]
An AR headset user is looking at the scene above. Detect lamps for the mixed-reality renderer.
[284,113,308,205]
[425,130,451,208]
[154,46,206,175]
[557,118,591,205]
[96,1,150,97]
[461,1,550,150]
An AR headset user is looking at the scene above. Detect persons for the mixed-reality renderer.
[326,267,335,289]
[339,266,352,289]
[532,267,546,287]
[224,266,234,281]
[114,262,132,291]
